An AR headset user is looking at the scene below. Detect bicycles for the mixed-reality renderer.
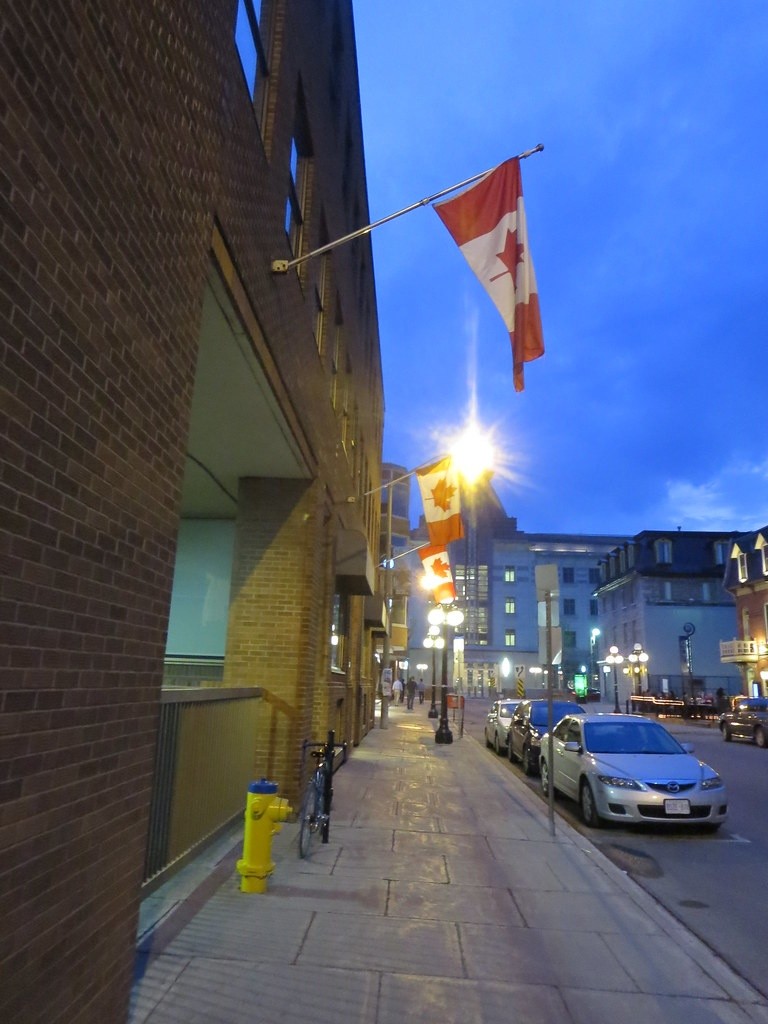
[295,739,348,858]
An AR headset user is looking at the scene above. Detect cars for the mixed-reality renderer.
[507,700,587,776]
[538,714,729,835]
[718,699,768,746]
[484,700,526,754]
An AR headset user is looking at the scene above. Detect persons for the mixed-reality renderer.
[678,692,692,719]
[417,678,425,704]
[406,676,417,710]
[699,692,709,720]
[642,688,676,718]
[392,678,405,707]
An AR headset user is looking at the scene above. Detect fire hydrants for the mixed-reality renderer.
[236,777,293,895]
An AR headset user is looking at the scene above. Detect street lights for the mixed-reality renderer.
[628,643,649,715]
[428,607,465,745]
[529,667,541,701]
[423,624,445,719]
[607,646,624,713]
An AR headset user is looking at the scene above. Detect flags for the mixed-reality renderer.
[432,157,545,390]
[417,545,456,605]
[419,451,466,542]
